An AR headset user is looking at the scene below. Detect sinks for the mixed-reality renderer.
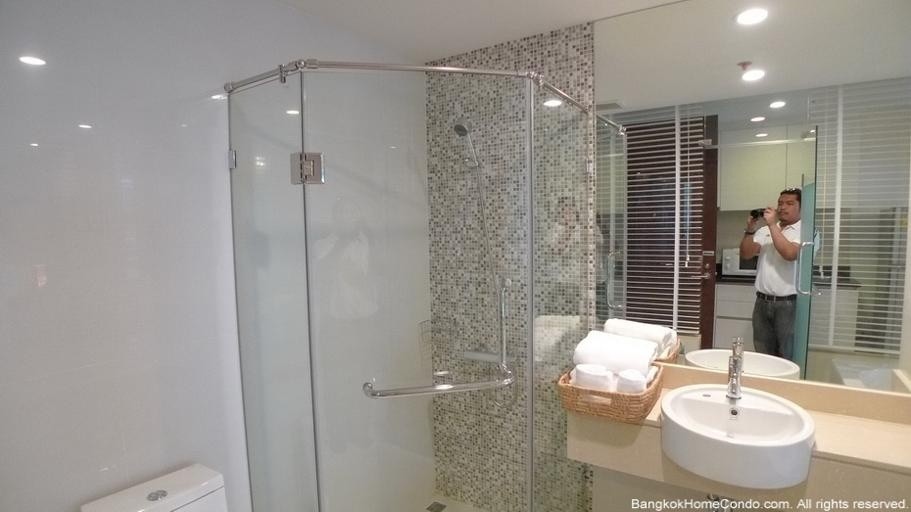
[659,383,816,490]
[684,348,801,381]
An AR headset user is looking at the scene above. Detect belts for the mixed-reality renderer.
[756,291,798,302]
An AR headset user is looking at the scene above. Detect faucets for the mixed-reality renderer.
[732,337,744,355]
[725,355,742,400]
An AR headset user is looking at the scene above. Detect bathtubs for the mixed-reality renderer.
[831,359,911,396]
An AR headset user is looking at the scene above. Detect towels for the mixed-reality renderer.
[571,331,660,393]
[604,318,678,356]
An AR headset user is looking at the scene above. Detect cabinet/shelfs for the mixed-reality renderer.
[719,124,816,211]
[713,280,860,349]
[591,467,762,511]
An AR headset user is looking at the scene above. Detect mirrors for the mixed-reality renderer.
[591,0,910,393]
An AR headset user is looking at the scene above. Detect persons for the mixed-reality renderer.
[544,197,606,320]
[740,188,820,361]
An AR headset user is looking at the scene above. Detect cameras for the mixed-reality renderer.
[751,209,764,218]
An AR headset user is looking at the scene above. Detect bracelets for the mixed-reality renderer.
[744,230,756,235]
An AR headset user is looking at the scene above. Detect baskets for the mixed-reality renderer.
[558,362,664,421]
[655,336,682,364]
